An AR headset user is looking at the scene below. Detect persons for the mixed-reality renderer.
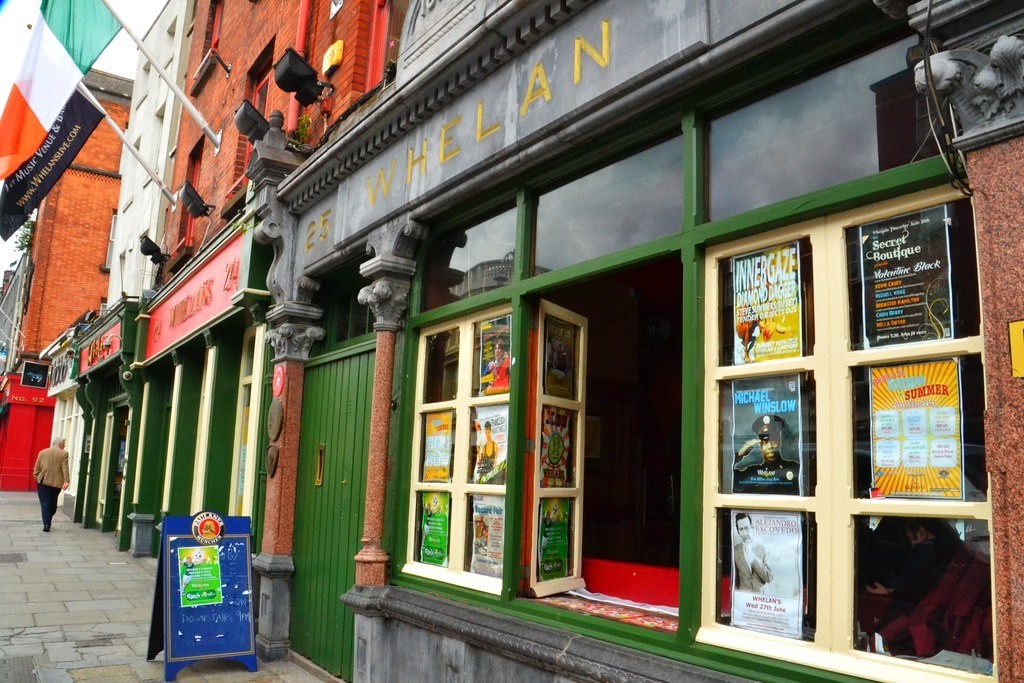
[475,516,488,545]
[549,340,567,376]
[540,510,559,561]
[480,332,511,393]
[734,512,774,594]
[468,406,498,483]
[737,260,775,364]
[33,438,70,532]
[420,503,439,563]
[732,414,800,495]
[180,557,204,595]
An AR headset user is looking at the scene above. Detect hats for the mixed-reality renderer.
[752,415,784,439]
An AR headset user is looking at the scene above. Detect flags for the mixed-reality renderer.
[1,0,122,181]
[0,89,105,242]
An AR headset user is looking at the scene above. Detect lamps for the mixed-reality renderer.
[139,234,170,264]
[181,181,216,218]
[233,99,272,145]
[271,46,334,105]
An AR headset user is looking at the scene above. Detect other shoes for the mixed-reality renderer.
[43,523,50,531]
